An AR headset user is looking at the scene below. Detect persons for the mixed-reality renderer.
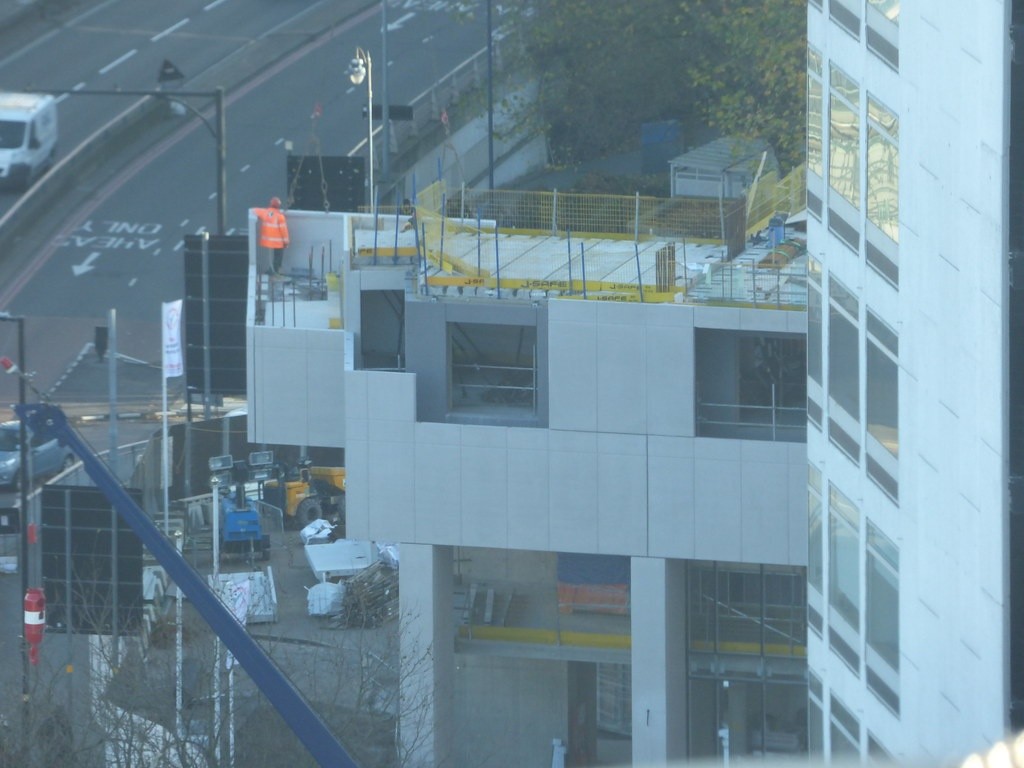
[256,198,290,273]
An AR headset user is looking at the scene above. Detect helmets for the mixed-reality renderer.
[270,197,282,207]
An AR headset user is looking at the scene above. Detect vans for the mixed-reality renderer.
[1,90,59,191]
[0,420,75,494]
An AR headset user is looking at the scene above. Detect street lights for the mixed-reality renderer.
[343,47,374,213]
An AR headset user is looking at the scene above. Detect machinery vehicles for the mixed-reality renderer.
[264,463,346,529]
[219,471,272,564]
